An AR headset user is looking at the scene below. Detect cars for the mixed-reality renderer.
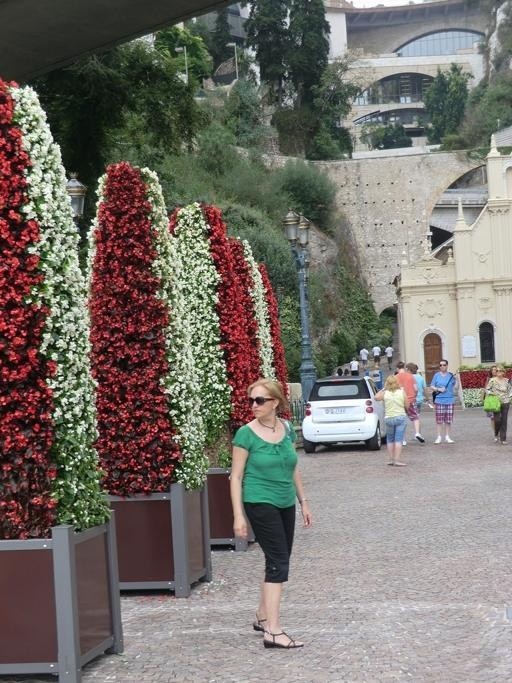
[300,376,386,452]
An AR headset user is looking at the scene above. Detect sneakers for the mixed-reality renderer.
[386,432,454,467]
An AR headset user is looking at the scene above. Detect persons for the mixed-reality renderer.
[335,367,350,375]
[229,377,313,649]
[359,346,369,368]
[373,361,426,467]
[371,344,381,367]
[384,344,394,371]
[482,364,512,445]
[349,357,360,377]
[430,359,465,445]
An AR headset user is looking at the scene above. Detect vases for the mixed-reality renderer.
[102,479,212,598]
[208,468,256,551]
[0,509,124,683]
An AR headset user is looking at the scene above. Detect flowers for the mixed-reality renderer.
[83,159,209,495]
[2,78,111,540]
[166,199,293,492]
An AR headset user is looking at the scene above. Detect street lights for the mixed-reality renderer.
[64,171,87,242]
[281,206,318,403]
[175,46,189,82]
[224,41,241,79]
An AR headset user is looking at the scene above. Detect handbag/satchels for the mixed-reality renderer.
[483,394,501,413]
[431,390,438,404]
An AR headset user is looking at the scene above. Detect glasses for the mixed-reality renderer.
[248,397,275,408]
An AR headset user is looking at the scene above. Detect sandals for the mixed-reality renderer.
[252,613,266,632]
[263,630,304,648]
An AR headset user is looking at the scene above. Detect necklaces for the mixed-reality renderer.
[257,416,277,432]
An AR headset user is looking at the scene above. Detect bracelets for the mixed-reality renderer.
[437,387,438,392]
[298,498,307,504]
